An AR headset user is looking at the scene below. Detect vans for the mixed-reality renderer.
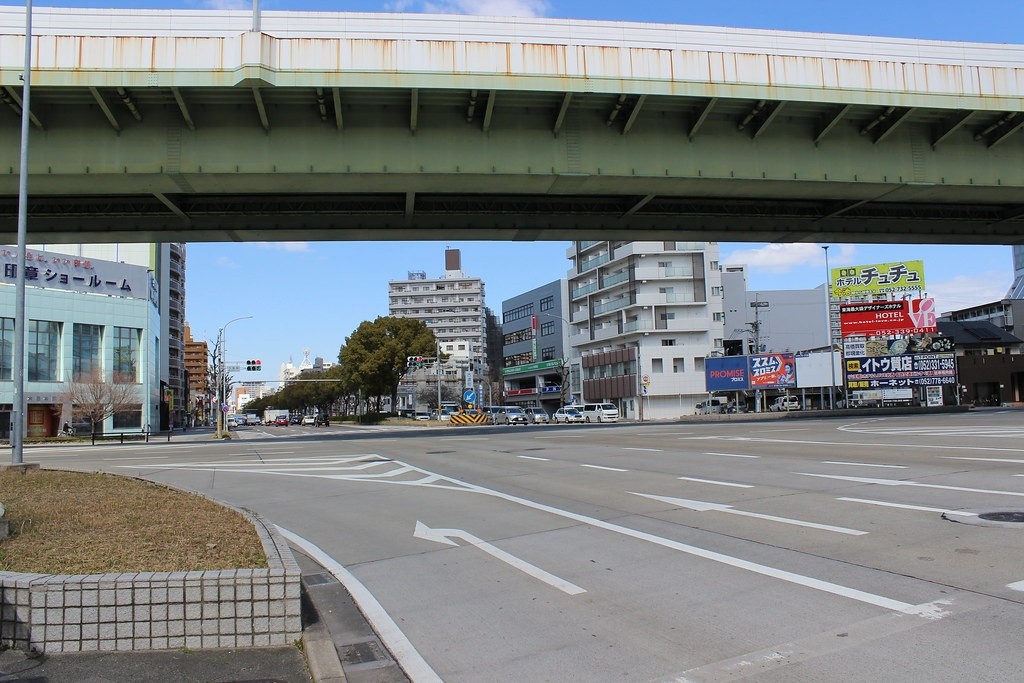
[400,404,501,424]
[695,399,748,415]
[846,399,881,408]
[564,405,585,418]
[524,407,550,424]
[582,402,619,423]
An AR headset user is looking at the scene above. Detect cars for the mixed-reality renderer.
[553,408,583,423]
[495,407,529,426]
[227,414,260,428]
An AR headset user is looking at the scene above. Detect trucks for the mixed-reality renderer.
[836,389,912,408]
[264,409,290,426]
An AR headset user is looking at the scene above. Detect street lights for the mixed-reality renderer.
[223,316,254,429]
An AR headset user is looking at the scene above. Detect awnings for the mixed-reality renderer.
[972,363,1003,384]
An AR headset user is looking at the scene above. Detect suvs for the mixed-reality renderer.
[276,415,288,427]
[770,396,800,412]
[290,415,329,428]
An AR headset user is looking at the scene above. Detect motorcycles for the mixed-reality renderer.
[58,427,76,437]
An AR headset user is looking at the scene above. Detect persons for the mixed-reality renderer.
[62,420,73,438]
[777,362,795,384]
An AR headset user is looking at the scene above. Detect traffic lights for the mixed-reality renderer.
[247,366,261,371]
[407,362,422,367]
[407,356,422,361]
[247,360,261,365]
